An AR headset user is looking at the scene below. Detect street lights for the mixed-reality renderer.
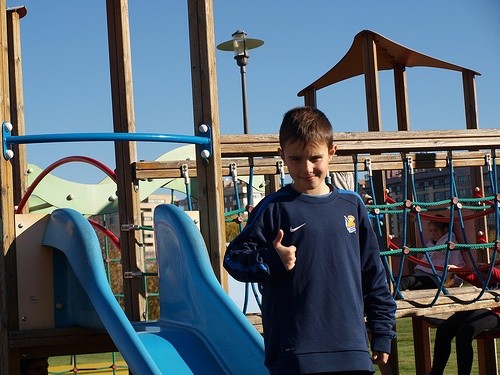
[216,29,264,134]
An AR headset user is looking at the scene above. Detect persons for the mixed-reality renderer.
[222,107,396,375]
[432,241,500,375]
[398,213,466,290]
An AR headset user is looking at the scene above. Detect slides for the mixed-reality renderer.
[40,202,285,375]
